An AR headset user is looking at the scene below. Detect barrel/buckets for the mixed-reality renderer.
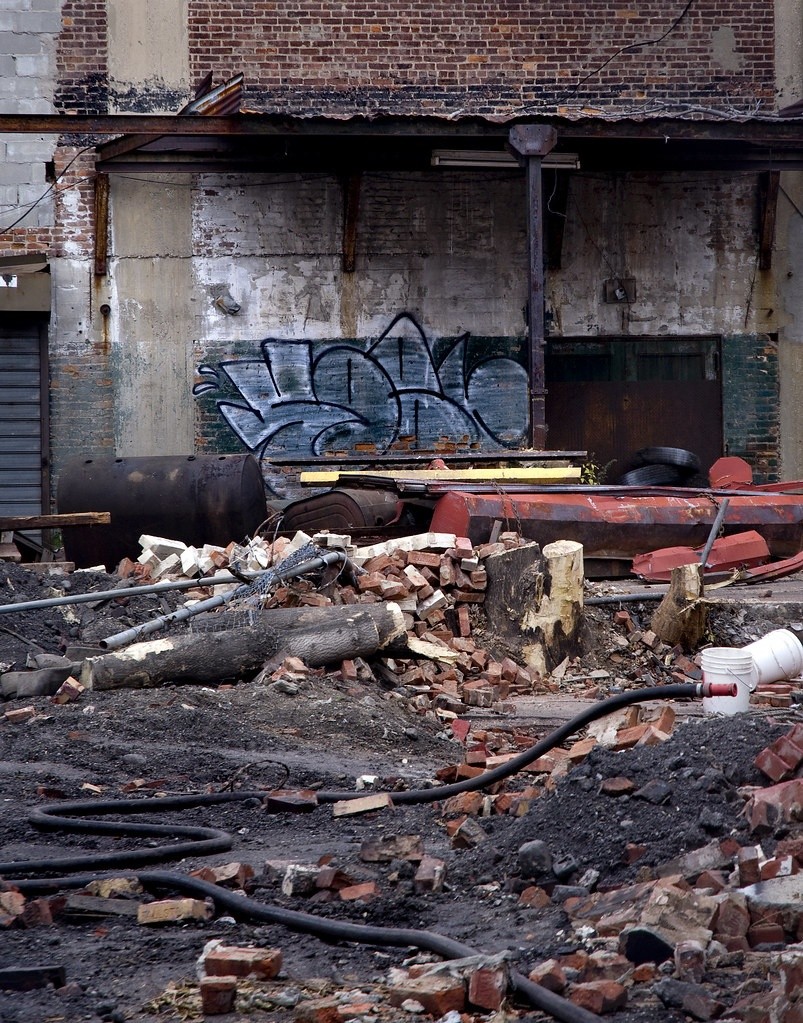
[283,488,402,530]
[740,629,803,691]
[701,647,753,715]
